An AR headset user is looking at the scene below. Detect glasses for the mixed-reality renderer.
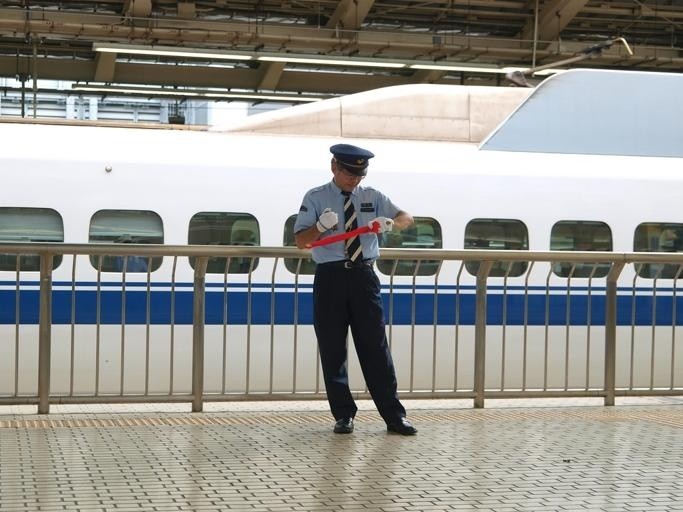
[72,81,340,102]
[92,35,569,90]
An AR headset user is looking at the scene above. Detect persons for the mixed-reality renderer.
[291,143,419,436]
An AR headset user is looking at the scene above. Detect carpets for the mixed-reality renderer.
[341,191,363,264]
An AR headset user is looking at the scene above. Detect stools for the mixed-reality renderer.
[320,260,373,267]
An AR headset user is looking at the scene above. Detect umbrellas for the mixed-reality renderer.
[334,417,354,432]
[387,417,418,434]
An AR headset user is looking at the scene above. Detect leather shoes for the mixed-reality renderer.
[316,208,338,233]
[368,217,394,234]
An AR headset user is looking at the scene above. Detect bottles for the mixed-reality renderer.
[330,144,374,175]
